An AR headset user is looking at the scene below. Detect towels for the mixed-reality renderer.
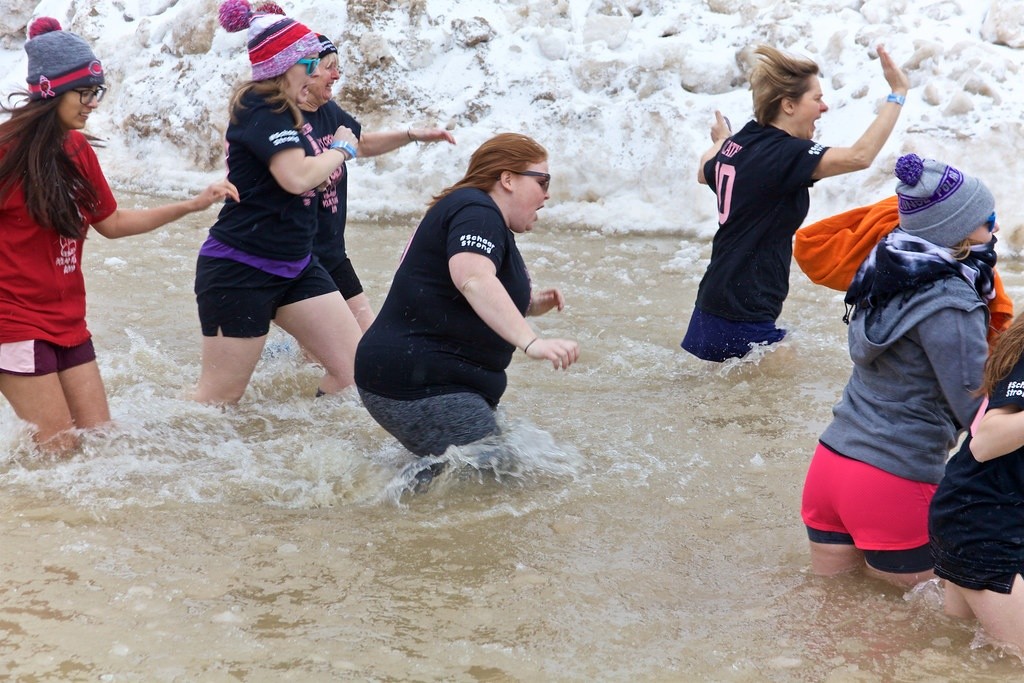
[792,196,1014,359]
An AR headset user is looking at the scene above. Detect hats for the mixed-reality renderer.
[217,1,322,82]
[256,2,338,57]
[893,155,994,248]
[23,17,106,99]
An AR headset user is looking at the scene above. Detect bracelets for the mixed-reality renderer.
[329,141,357,158]
[523,337,540,354]
[406,128,417,144]
[887,94,906,106]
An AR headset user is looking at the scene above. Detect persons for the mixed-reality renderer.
[926,308,1024,655]
[801,154,1001,587]
[0,16,241,460]
[311,34,458,335]
[354,133,585,507]
[192,0,365,407]
[681,43,912,369]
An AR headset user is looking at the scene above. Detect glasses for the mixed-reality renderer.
[295,56,321,75]
[73,86,108,105]
[496,167,551,191]
[987,213,996,231]
